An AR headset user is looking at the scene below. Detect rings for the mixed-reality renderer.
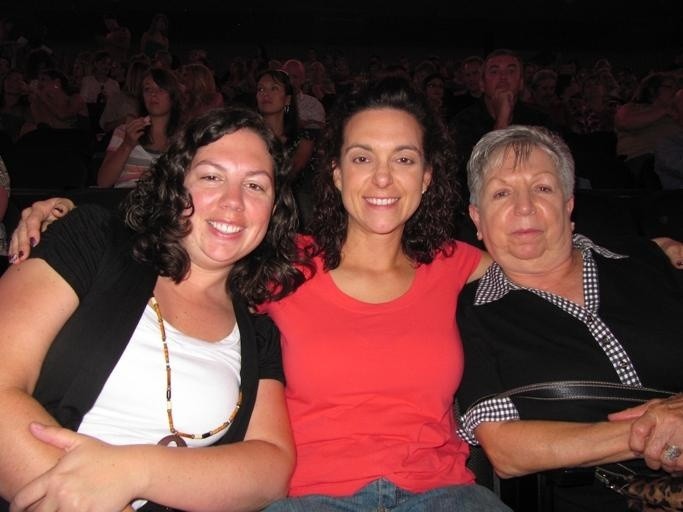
[662,443,682,461]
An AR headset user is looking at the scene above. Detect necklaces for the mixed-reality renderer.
[151,296,242,447]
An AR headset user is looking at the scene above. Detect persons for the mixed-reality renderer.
[0,12,683,269]
[1,107,307,511]
[6,83,682,511]
[453,125,683,511]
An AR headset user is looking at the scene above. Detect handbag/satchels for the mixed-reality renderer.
[464,380,683,512]
